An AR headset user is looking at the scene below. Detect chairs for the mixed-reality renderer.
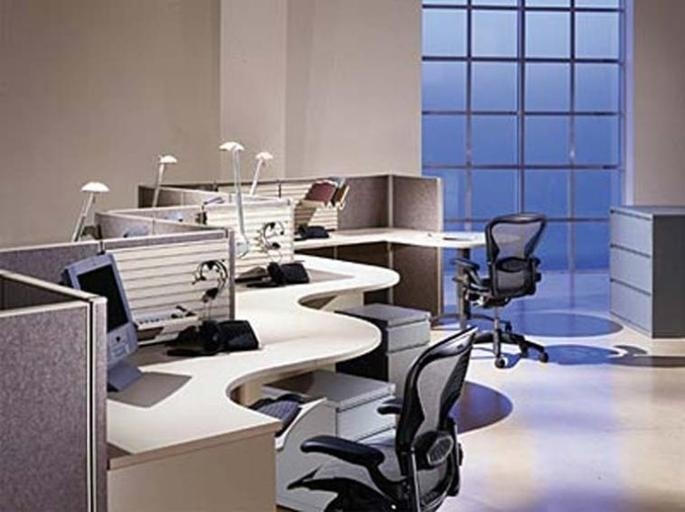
[450,211,550,367]
[285,325,479,511]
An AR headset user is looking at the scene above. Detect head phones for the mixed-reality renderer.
[262,219,284,250]
[192,259,229,300]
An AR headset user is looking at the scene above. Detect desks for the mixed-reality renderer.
[107,223,518,512]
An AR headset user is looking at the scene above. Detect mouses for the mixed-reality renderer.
[276,393,305,403]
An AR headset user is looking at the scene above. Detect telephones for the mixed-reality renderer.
[200,319,258,351]
[299,224,329,238]
[268,263,310,284]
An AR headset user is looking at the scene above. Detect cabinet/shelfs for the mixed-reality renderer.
[263,367,398,512]
[610,208,684,337]
[333,302,432,408]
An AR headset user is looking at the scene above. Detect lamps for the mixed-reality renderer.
[69,139,274,255]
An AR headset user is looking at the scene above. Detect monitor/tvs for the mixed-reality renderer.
[60,252,144,393]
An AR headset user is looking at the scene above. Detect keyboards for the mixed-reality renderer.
[248,398,300,437]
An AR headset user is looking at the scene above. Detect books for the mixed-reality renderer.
[327,174,347,206]
[336,181,351,206]
[301,179,338,207]
